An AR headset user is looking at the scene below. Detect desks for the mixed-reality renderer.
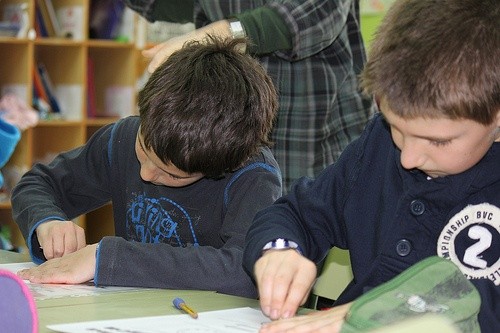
[0,249,318,333]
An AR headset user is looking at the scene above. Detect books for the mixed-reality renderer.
[33,61,64,117]
[35,0,63,38]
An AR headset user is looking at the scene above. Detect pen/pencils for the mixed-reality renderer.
[173,297,198,318]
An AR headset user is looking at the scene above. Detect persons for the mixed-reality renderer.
[11,30,283,299]
[126,0,379,195]
[0,90,38,188]
[241,0,500,333]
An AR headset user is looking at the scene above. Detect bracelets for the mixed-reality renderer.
[226,17,247,54]
[263,238,304,255]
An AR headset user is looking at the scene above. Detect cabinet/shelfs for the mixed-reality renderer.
[0,0,158,252]
